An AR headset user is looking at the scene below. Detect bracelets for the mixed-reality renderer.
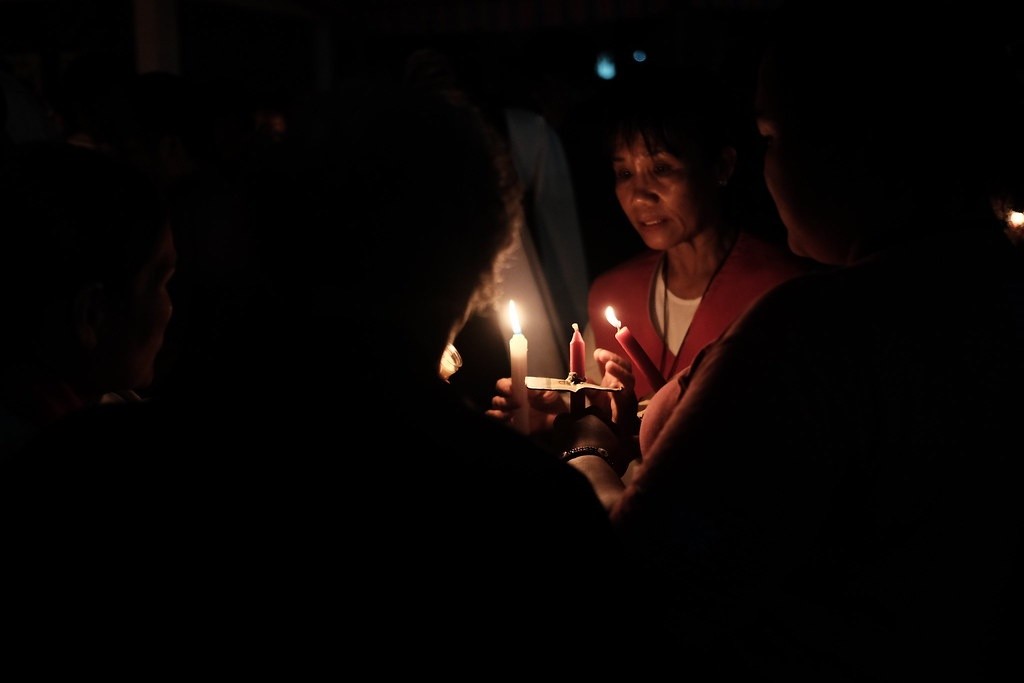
[559,445,615,468]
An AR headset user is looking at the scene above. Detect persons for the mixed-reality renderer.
[484,75,800,476]
[130,75,618,683]
[544,38,1024,683]
[0,156,188,683]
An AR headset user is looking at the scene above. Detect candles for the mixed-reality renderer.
[605,306,667,393]
[509,299,530,437]
[569,323,587,421]
